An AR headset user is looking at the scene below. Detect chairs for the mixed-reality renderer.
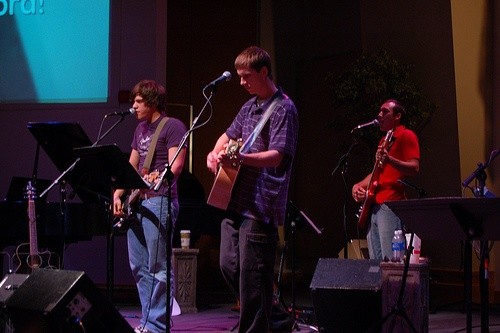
[427,238,473,333]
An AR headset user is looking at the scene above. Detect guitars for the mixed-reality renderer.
[15,180,54,274]
[208,137,243,210]
[357,128,393,229]
[113,169,173,231]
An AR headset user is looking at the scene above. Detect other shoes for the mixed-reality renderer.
[134,323,164,333]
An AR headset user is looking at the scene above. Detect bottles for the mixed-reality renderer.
[398,230,405,261]
[392,230,402,263]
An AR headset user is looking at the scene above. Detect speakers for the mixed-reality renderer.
[309,257,385,332]
[0,273,30,333]
[4,268,135,333]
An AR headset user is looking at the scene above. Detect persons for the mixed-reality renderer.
[206,46,299,333]
[352,99,420,261]
[110,80,187,333]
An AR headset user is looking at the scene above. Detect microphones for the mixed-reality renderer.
[352,118,380,132]
[203,71,232,90]
[106,108,136,117]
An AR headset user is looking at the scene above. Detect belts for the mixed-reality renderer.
[139,192,162,198]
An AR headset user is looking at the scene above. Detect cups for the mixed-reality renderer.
[180,230,191,249]
[405,233,421,264]
